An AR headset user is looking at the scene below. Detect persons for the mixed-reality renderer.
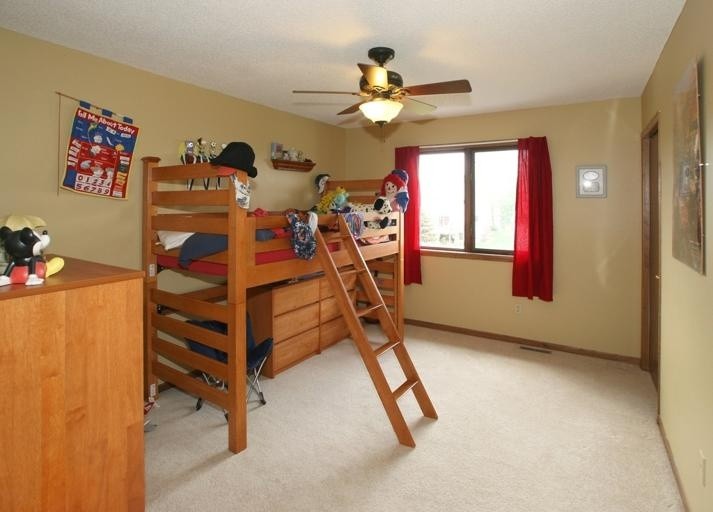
[196,136,211,190]
[208,140,221,190]
[181,139,197,191]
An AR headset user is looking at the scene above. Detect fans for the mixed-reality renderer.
[292,46,472,116]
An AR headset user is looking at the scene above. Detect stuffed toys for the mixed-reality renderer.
[309,169,409,243]
[272,147,313,162]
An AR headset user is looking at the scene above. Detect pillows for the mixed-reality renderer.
[155,229,194,252]
[179,228,275,269]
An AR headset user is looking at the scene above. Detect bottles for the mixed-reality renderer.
[275,143,282,160]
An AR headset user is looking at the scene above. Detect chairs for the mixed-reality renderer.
[184,313,273,423]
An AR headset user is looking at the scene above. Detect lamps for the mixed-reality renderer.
[359,97,403,129]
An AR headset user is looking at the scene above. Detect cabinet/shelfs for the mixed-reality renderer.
[0,253,146,512]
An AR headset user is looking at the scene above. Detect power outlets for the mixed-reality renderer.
[514,304,522,313]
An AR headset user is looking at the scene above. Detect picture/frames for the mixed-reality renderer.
[576,166,607,198]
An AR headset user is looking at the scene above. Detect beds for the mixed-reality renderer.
[142,156,438,453]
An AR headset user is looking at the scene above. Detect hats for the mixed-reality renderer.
[209,140,259,177]
